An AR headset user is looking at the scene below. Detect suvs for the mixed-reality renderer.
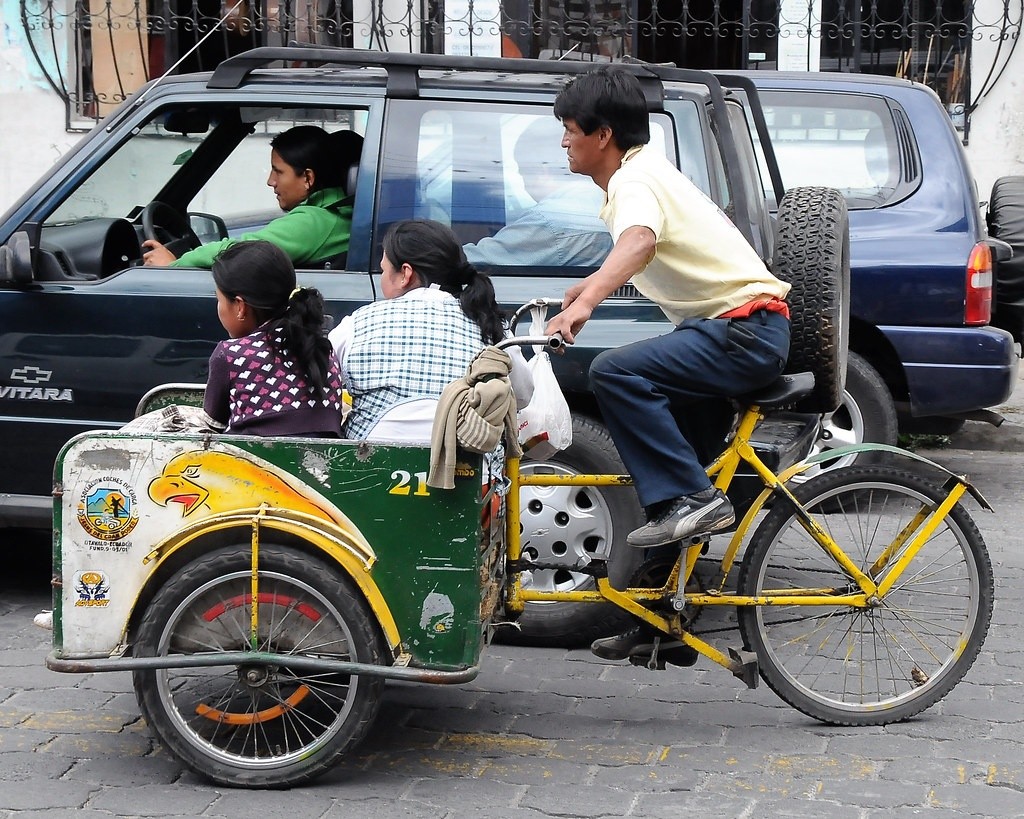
[0,43,851,644]
[220,69,1024,514]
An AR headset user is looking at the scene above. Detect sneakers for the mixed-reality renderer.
[33,612,52,631]
[626,488,736,548]
[590,625,686,660]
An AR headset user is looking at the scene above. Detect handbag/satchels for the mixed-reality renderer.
[501,296,572,461]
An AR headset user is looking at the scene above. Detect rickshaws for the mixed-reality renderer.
[43,296,997,791]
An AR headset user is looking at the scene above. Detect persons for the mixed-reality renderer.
[541,69,795,668]
[326,217,535,454]
[34,240,344,631]
[459,117,617,269]
[141,125,354,273]
